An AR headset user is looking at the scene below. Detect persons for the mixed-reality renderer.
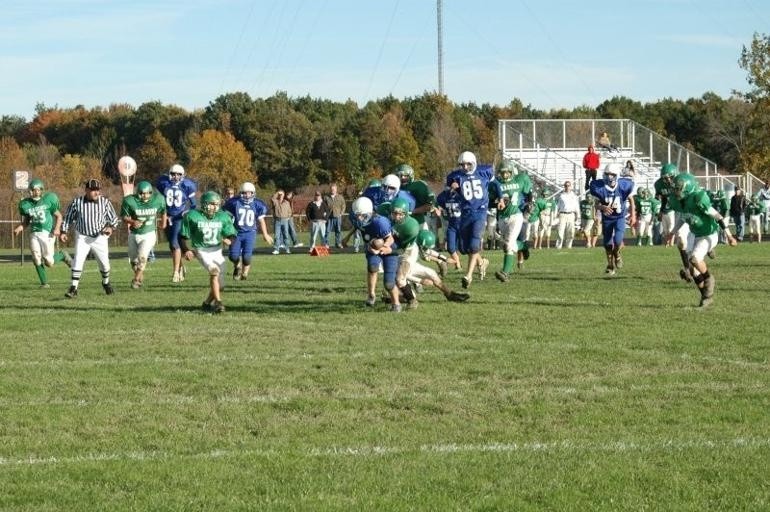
[305,184,346,252]
[224,186,235,200]
[271,189,304,254]
[14,178,74,287]
[221,182,276,282]
[156,164,197,284]
[341,150,581,312]
[179,190,238,312]
[122,181,167,288]
[599,132,620,154]
[579,146,769,308]
[58,178,119,298]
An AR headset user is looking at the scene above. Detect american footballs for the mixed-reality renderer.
[370,238,384,250]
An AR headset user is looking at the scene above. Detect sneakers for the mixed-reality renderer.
[172,267,186,283]
[231,264,246,280]
[495,271,508,282]
[272,243,303,255]
[518,249,529,270]
[606,250,622,273]
[479,259,489,280]
[39,282,49,288]
[131,279,143,289]
[438,255,461,278]
[202,300,224,312]
[444,291,469,301]
[366,284,424,312]
[102,279,113,294]
[63,250,72,268]
[680,250,714,308]
[65,287,77,298]
[462,276,472,288]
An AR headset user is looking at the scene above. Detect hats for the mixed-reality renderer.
[86,180,101,190]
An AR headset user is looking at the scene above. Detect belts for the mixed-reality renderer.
[560,211,573,214]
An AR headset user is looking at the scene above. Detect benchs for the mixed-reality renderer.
[503,147,663,228]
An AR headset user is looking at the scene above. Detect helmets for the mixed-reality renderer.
[418,230,436,249]
[169,164,185,184]
[238,183,255,204]
[29,180,44,201]
[201,192,221,219]
[137,181,153,202]
[661,164,697,200]
[458,151,476,175]
[497,160,514,183]
[602,162,618,183]
[352,163,414,226]
